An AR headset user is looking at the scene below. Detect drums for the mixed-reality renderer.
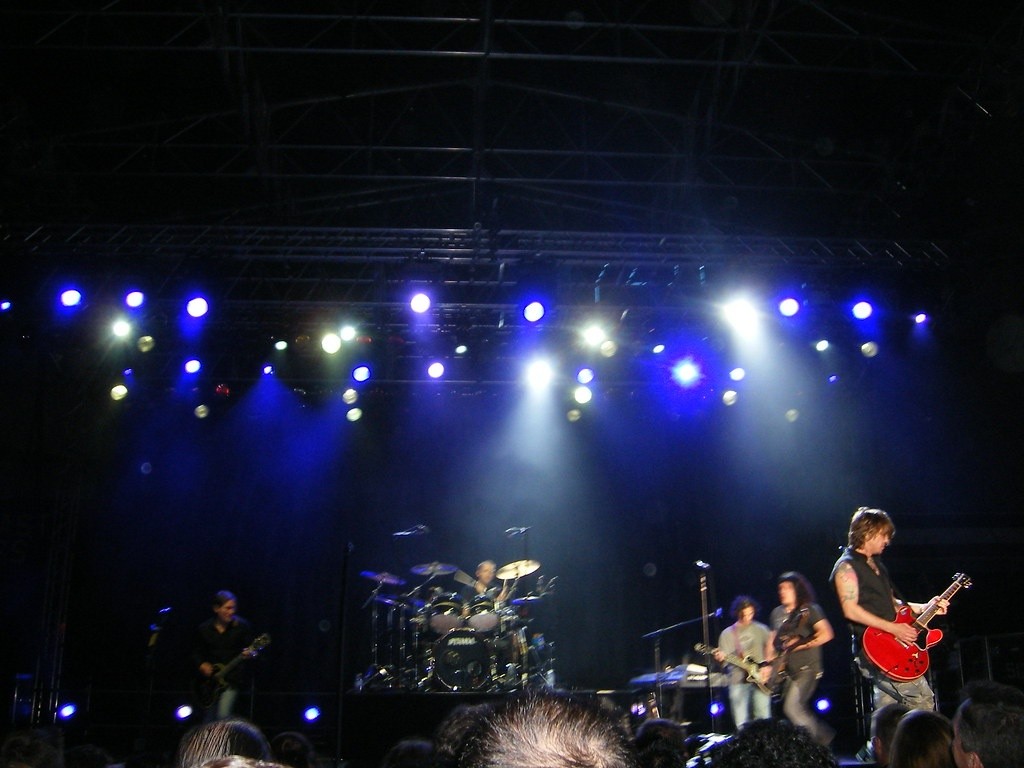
[466,594,498,632]
[428,591,464,634]
[431,627,494,691]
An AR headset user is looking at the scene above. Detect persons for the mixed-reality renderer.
[192,591,252,722]
[828,507,951,712]
[0,680,1024,768]
[759,571,835,745]
[460,559,499,599]
[713,596,774,732]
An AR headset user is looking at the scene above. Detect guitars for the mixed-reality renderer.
[694,642,774,696]
[193,633,272,710]
[860,571,973,684]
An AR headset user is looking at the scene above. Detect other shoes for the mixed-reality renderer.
[821,730,836,747]
[855,745,872,762]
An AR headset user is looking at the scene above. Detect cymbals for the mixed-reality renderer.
[410,563,458,575]
[496,559,541,580]
[360,570,406,585]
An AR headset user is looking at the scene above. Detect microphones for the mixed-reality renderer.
[694,560,711,571]
[392,524,431,539]
[505,526,535,538]
[159,607,173,614]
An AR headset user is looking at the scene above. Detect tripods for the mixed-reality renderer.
[346,572,553,691]
